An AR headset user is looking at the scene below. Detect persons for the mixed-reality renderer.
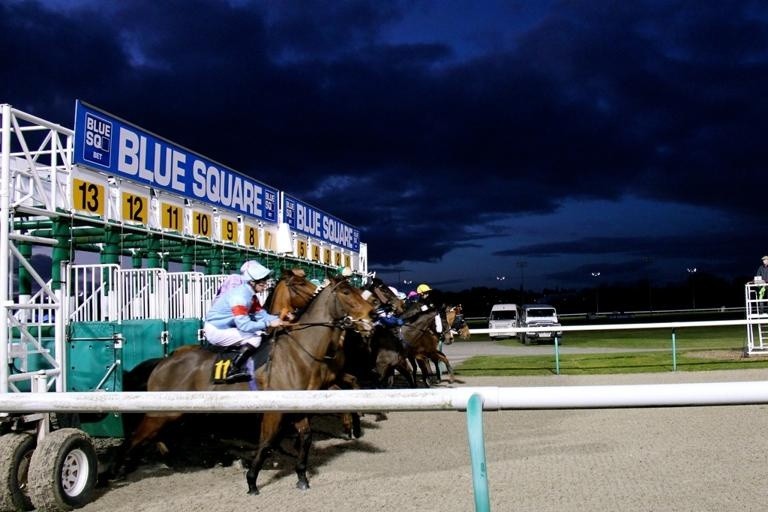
[756,255,768,314]
[213,259,256,299]
[310,265,464,338]
[204,265,283,385]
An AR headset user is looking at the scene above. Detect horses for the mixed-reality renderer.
[93,267,472,499]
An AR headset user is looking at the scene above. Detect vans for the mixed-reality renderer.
[487,303,521,342]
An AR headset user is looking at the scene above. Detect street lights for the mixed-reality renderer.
[496,275,507,303]
[515,259,528,291]
[402,279,412,293]
[686,267,696,309]
[591,271,601,313]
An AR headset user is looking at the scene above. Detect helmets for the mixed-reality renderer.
[243,261,271,281]
[417,284,432,294]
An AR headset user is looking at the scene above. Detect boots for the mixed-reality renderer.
[225,343,253,379]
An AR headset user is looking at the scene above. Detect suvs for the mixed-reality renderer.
[518,303,563,346]
